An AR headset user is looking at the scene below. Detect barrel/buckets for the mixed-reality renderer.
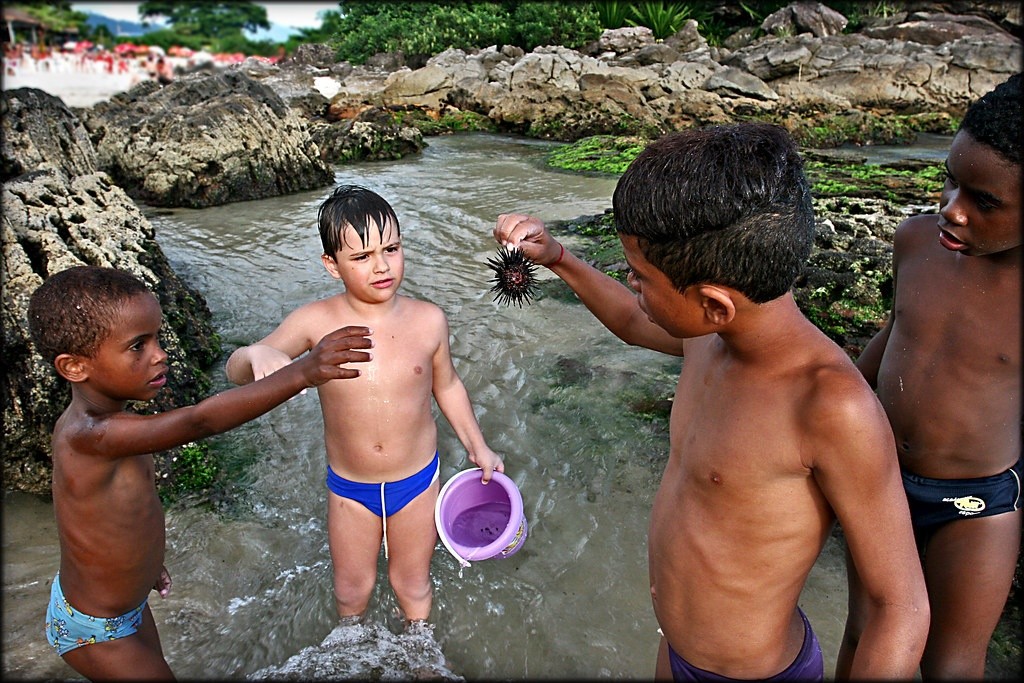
[435,467,528,568]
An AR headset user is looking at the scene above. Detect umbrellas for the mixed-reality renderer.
[61,39,279,65]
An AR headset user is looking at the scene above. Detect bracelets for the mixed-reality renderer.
[544,243,565,269]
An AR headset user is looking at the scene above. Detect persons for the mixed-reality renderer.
[492,117,930,683]
[2,45,171,84]
[828,74,1024,683]
[226,184,506,624]
[26,265,378,683]
